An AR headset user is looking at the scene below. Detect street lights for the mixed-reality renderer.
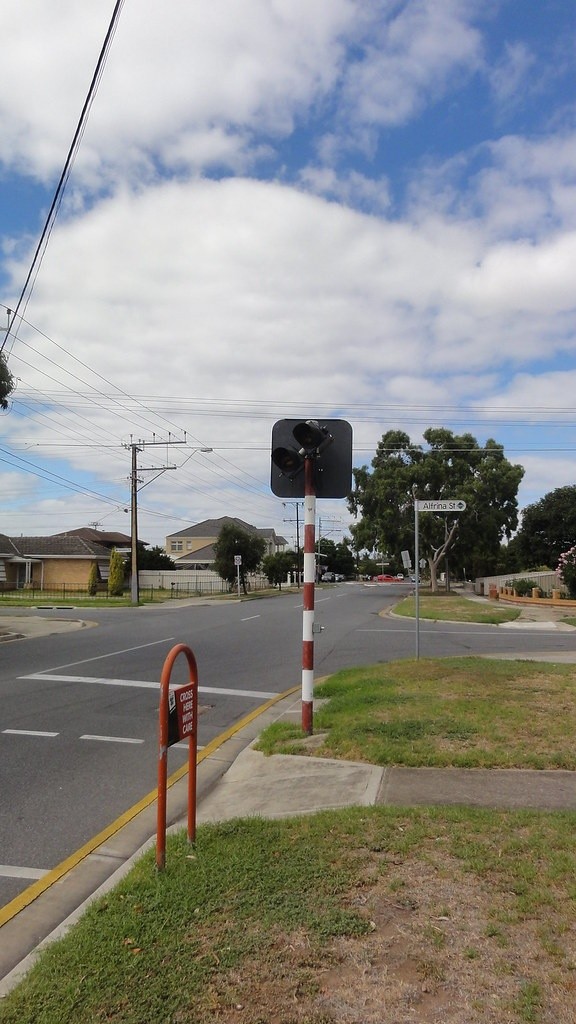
[130,447,215,606]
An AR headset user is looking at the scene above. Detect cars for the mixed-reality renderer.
[322,572,421,584]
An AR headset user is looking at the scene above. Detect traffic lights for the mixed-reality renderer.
[271,420,334,488]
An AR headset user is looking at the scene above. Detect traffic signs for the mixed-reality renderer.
[414,499,467,514]
